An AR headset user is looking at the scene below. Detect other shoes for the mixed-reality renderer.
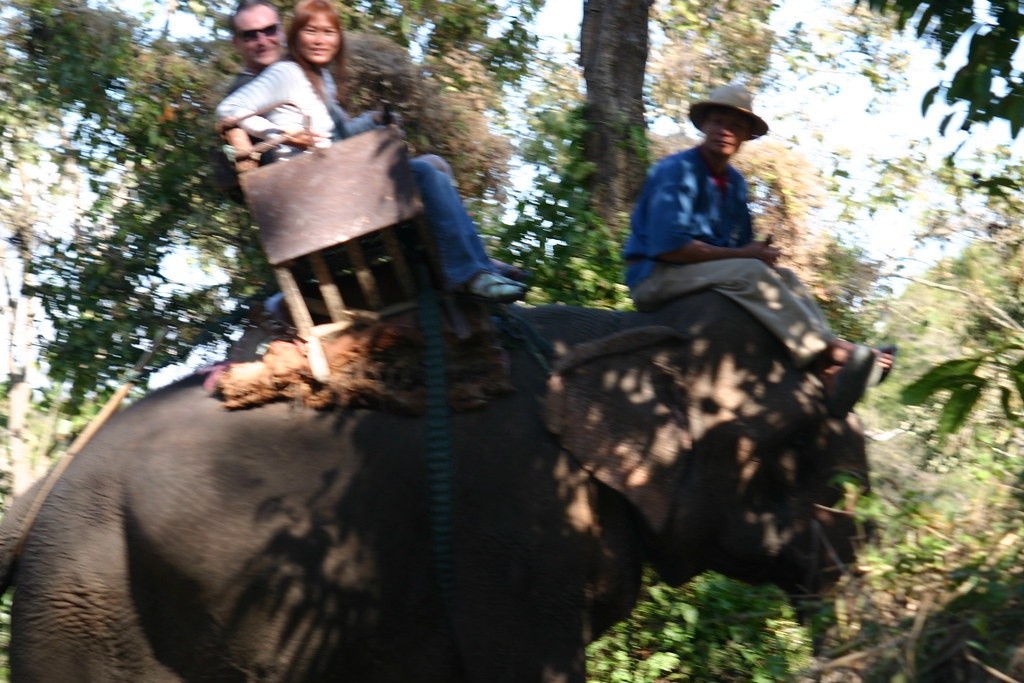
[448,273,532,309]
[478,253,530,282]
[818,341,897,417]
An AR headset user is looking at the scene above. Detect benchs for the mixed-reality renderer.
[224,125,427,384]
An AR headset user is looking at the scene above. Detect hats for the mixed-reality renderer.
[688,86,769,141]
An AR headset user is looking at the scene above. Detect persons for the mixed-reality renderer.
[215,0,530,300]
[620,84,897,387]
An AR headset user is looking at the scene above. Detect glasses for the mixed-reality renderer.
[237,24,282,42]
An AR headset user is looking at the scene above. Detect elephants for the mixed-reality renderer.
[0,287,885,683]
